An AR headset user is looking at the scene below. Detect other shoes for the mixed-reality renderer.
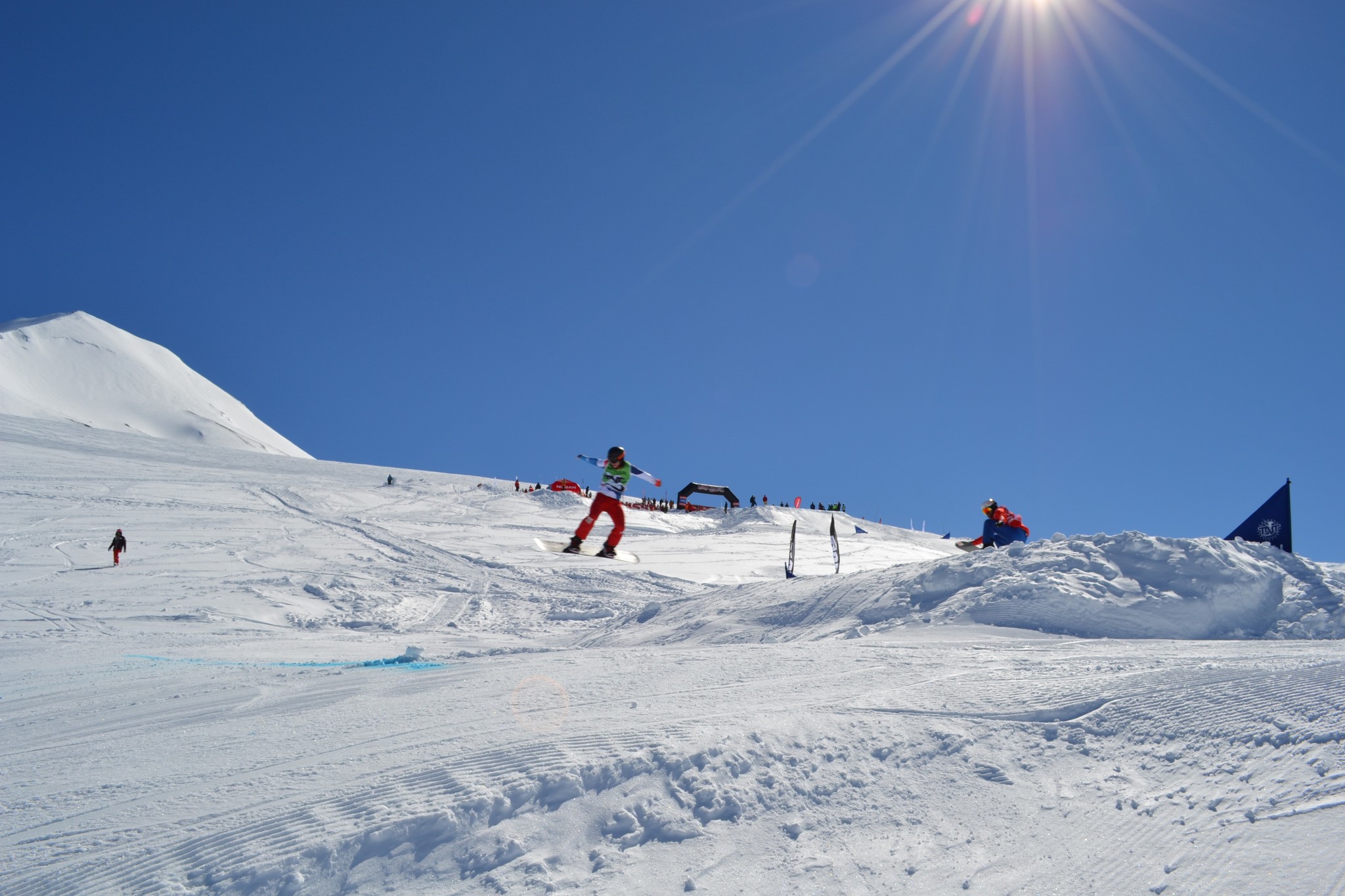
[603,546,617,558]
[567,544,580,553]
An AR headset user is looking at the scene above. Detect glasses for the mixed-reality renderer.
[982,508,992,514]
[608,456,618,463]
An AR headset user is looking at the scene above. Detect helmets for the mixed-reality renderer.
[981,499,997,509]
[607,447,625,460]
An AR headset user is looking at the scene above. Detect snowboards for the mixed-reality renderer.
[955,541,981,552]
[533,538,642,564]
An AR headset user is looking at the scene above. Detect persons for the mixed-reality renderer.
[585,486,589,497]
[763,495,767,505]
[387,474,393,485]
[785,503,789,507]
[561,478,565,490]
[724,502,728,514]
[810,502,815,510]
[546,484,552,490]
[642,496,674,509]
[750,495,757,508]
[108,529,126,565]
[970,499,1029,550]
[818,501,845,512]
[780,502,784,507]
[535,482,541,489]
[588,492,591,498]
[568,446,662,556]
[524,488,527,493]
[515,479,519,491]
[529,485,534,492]
[685,500,692,513]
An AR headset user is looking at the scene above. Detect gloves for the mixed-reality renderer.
[576,455,582,459]
[655,479,662,487]
